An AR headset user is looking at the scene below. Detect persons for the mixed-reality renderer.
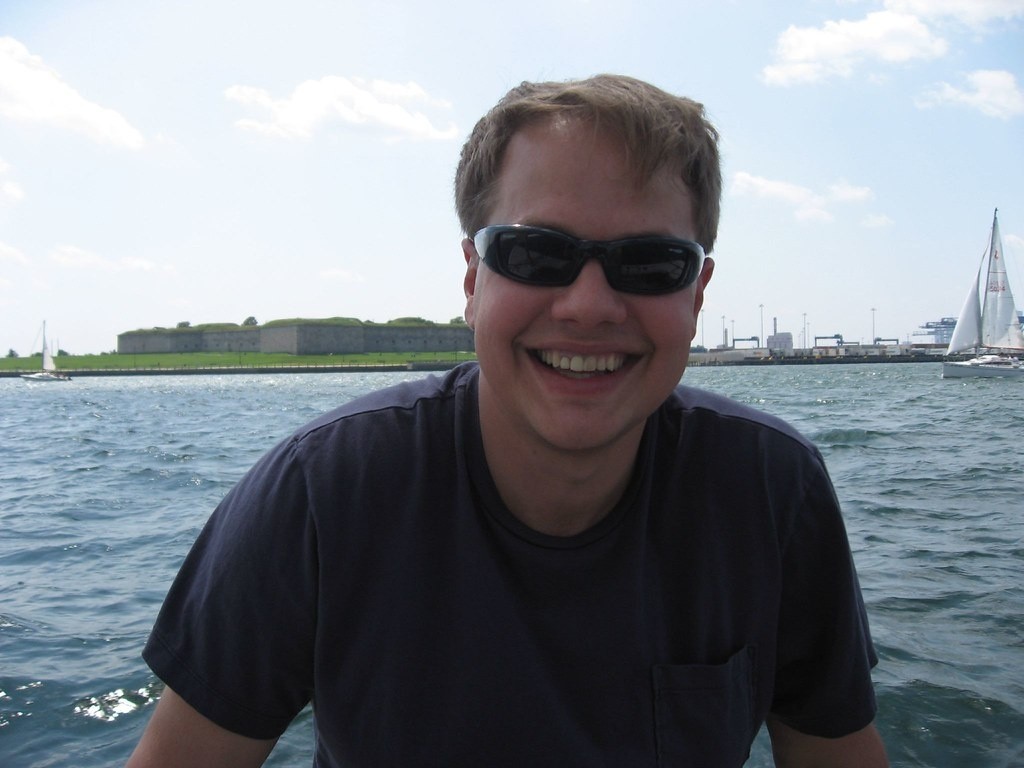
[123,75,891,768]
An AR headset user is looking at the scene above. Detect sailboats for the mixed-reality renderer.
[19,321,73,380]
[942,208,1024,379]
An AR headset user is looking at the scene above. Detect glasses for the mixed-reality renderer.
[470,224,707,298]
